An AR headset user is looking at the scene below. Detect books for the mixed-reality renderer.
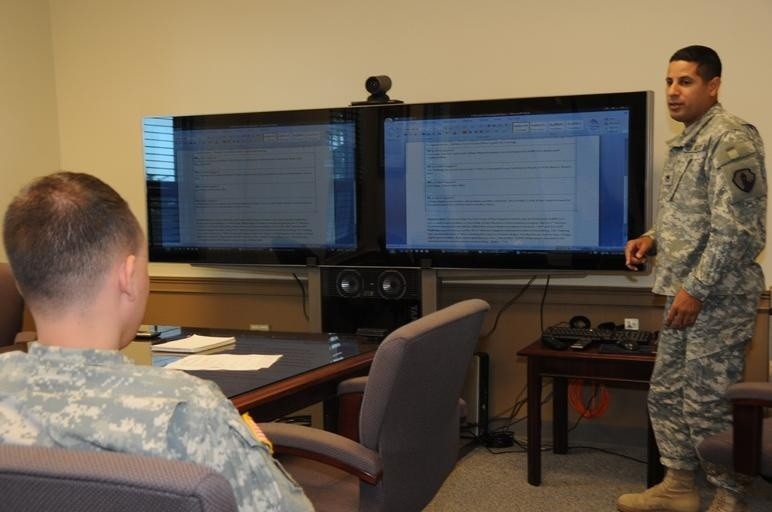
[152,331,236,354]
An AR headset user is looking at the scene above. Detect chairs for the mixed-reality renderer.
[0,261,27,345]
[0,439,241,512]
[240,295,492,511]
[697,379,771,495]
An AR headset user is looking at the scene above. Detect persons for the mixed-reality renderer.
[0,168,317,512]
[617,43,768,512]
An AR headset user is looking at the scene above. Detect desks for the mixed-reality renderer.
[0,323,381,426]
[517,338,662,493]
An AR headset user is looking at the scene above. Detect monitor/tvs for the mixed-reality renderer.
[144,107,373,266]
[372,90,654,272]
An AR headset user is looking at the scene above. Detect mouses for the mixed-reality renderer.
[616,340,639,351]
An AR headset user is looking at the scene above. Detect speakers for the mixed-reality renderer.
[320,266,437,335]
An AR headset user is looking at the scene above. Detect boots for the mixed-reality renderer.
[706,488,753,512]
[616,468,703,512]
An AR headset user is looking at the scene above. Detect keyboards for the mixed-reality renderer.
[542,326,652,344]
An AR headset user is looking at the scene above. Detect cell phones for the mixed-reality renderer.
[136,331,161,339]
[570,340,592,349]
